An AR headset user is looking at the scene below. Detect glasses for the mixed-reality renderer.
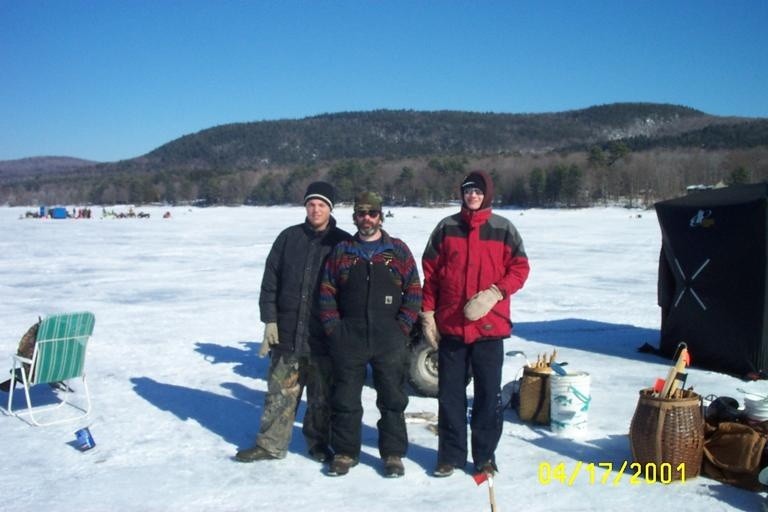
[464,190,483,194]
[357,212,378,218]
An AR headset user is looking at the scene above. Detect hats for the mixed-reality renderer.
[354,190,383,211]
[462,175,485,191]
[701,396,745,426]
[304,182,334,212]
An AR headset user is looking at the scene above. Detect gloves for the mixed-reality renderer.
[464,284,503,321]
[260,323,280,358]
[419,309,442,351]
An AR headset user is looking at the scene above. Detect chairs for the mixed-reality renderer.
[0,311,96,427]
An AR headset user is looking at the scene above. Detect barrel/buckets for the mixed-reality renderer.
[548,369,591,435]
[736,387,768,421]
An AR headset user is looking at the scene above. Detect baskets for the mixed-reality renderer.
[518,364,551,424]
[629,385,702,483]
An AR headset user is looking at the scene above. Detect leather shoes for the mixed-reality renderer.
[236,446,273,462]
[476,461,493,480]
[310,444,334,462]
[432,464,453,477]
[383,455,405,478]
[328,454,359,476]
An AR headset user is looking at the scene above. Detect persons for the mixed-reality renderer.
[420,170,530,479]
[72,207,172,219]
[235,182,353,463]
[318,191,423,478]
